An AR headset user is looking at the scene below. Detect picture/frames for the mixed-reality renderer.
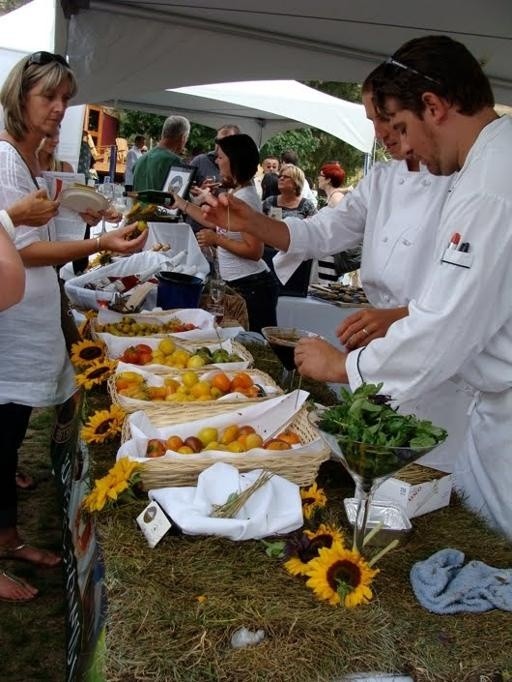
[156,165,197,220]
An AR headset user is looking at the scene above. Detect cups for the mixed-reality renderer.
[261,326,325,374]
[306,405,447,554]
[96,181,114,235]
[204,304,224,323]
[209,279,227,303]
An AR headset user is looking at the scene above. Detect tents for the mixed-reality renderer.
[99,79,376,174]
[0,0,511,172]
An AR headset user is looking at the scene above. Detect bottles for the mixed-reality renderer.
[100,264,162,293]
[158,249,188,272]
[122,266,184,297]
[83,277,121,290]
[122,191,175,207]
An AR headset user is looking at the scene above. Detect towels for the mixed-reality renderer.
[410,547,512,614]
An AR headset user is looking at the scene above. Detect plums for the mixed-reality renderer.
[115,369,260,403]
[117,338,244,371]
[144,425,302,460]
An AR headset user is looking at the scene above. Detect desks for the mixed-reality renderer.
[277,284,366,393]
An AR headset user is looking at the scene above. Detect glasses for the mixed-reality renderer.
[22,50,74,72]
[278,173,293,180]
[318,173,326,179]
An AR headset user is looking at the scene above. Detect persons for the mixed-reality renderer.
[0,189,59,313]
[201,59,460,352]
[262,173,280,202]
[189,124,240,188]
[133,116,190,208]
[0,52,149,602]
[254,155,279,198]
[317,161,363,289]
[35,122,74,179]
[280,150,317,208]
[78,131,91,186]
[295,36,512,538]
[125,135,145,192]
[162,134,279,332]
[262,164,318,298]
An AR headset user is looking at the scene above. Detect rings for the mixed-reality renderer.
[363,329,369,336]
[367,331,369,334]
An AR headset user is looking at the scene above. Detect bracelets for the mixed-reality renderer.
[96,236,100,249]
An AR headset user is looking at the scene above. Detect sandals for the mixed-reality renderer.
[0,542,64,570]
[0,569,39,606]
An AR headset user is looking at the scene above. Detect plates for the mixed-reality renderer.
[58,187,107,214]
